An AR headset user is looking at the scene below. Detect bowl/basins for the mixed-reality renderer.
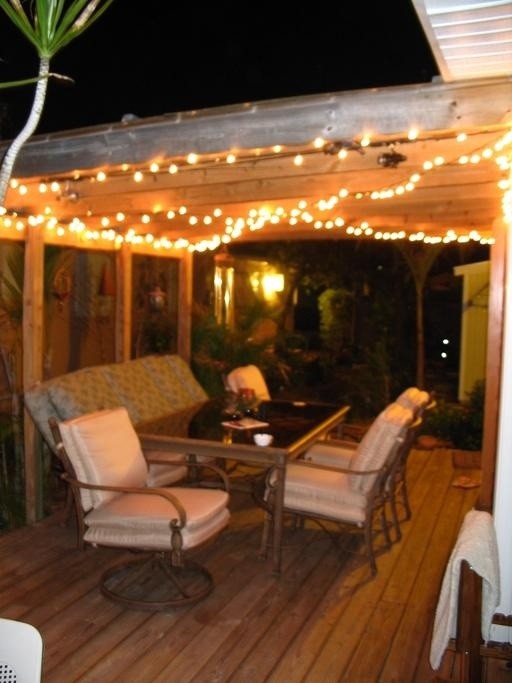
[253,433,273,447]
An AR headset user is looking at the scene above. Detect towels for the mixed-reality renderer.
[429,510,501,670]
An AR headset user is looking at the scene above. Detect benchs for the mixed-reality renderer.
[24,355,226,550]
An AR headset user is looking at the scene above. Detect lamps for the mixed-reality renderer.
[149,287,167,312]
[272,274,285,292]
[53,276,71,313]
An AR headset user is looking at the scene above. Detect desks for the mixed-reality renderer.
[131,393,351,581]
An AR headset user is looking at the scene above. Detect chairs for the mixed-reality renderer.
[456,560,511,682]
[47,406,232,607]
[260,402,419,578]
[0,618,43,683]
[297,387,437,542]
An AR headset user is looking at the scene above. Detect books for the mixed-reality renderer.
[221,413,270,431]
